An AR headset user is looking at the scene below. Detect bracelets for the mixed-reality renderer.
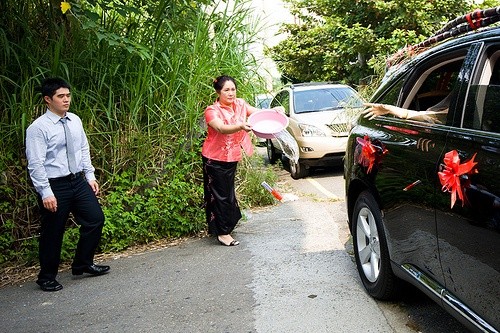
[402,109,408,119]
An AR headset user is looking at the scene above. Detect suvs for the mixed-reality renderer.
[342,25,500,333]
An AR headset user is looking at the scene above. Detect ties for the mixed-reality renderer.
[59,119,78,174]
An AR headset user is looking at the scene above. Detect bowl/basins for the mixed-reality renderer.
[247,109,289,139]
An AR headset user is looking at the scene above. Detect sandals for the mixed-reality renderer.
[217,234,240,246]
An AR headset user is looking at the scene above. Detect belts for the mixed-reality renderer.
[59,172,81,182]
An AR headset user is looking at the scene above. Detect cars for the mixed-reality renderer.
[266,81,370,179]
[254,93,275,147]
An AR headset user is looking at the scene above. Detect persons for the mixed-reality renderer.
[361,94,453,120]
[27,76,111,292]
[199,76,285,246]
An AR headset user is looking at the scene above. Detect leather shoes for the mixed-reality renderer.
[36,279,63,291]
[71,264,109,275]
